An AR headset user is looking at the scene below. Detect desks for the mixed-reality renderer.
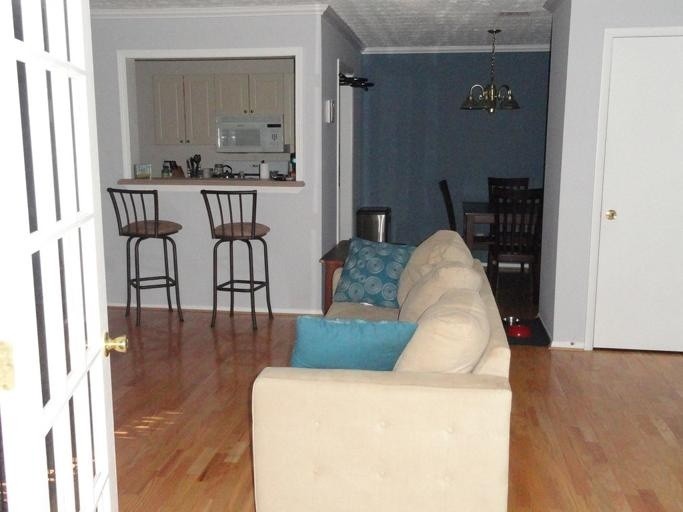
[319,240,408,316]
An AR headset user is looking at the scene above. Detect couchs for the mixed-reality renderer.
[252,229,512,512]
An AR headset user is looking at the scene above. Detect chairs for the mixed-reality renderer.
[200,189,274,331]
[107,187,184,327]
[439,176,544,315]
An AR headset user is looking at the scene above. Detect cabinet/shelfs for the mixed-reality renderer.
[152,74,212,146]
[213,72,294,146]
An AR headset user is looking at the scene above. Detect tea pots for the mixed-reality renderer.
[220,165,234,179]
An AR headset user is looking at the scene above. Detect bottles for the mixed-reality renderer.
[162,161,171,172]
[161,166,170,177]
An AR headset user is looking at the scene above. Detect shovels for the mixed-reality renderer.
[194,155,201,177]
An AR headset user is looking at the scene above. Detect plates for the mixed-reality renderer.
[507,325,531,338]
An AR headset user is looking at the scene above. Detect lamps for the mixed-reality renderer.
[460,28,521,115]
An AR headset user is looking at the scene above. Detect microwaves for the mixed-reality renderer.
[217,113,285,153]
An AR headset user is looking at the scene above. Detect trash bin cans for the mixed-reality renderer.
[357,207,391,242]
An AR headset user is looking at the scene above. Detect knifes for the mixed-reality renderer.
[169,161,178,170]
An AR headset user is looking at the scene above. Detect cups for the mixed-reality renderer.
[203,168,212,177]
[214,164,223,175]
[259,164,269,179]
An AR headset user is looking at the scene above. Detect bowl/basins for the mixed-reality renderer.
[502,316,519,324]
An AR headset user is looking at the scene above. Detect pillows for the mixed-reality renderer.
[332,236,417,308]
[290,314,418,371]
[398,260,484,322]
[398,229,473,309]
[392,287,491,374]
